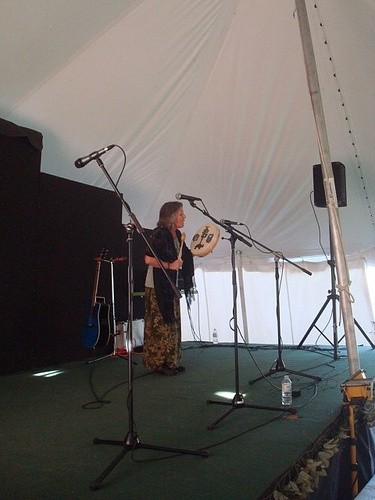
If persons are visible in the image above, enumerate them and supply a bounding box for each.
[143,201,186,376]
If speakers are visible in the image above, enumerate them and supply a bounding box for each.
[313,161,347,207]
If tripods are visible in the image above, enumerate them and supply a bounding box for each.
[91,157,375,492]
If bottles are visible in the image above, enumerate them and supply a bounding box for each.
[213,328,218,344]
[282,374,292,406]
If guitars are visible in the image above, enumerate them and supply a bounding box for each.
[82,247,110,349]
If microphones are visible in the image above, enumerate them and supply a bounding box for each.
[75,144,116,168]
[220,219,245,225]
[175,193,201,201]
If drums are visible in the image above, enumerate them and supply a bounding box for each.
[190,224,220,257]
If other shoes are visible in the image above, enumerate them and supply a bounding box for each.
[176,366,185,372]
[157,364,175,376]
